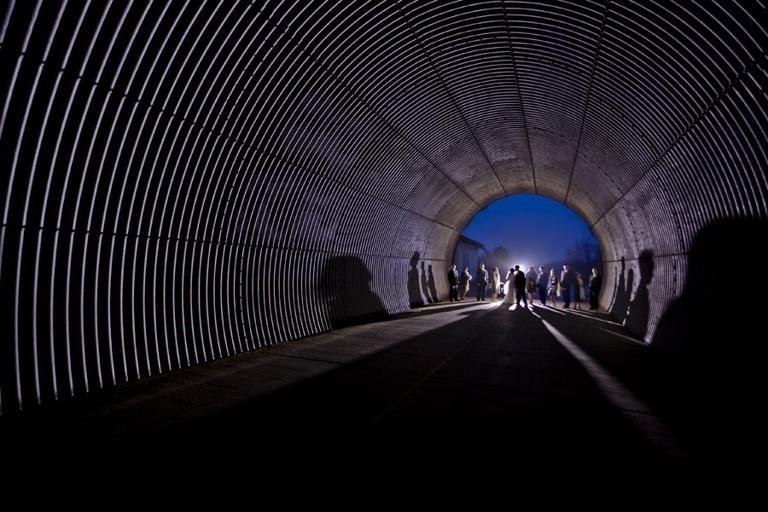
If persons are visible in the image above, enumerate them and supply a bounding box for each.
[448,262,602,311]
